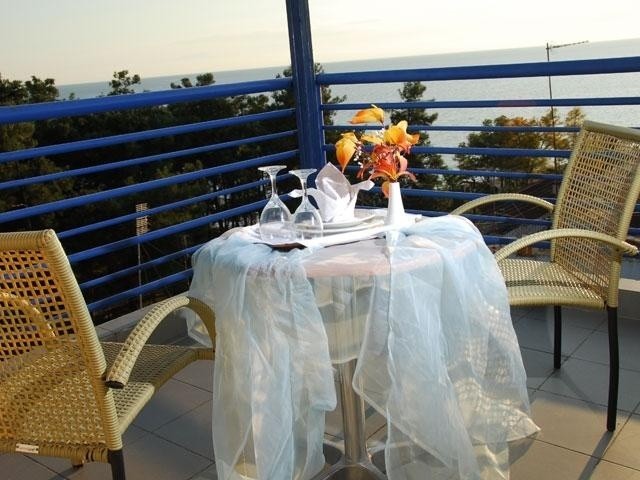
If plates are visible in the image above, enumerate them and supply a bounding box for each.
[296,210,377,228]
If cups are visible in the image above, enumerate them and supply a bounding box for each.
[288,168,323,241]
[257,165,293,242]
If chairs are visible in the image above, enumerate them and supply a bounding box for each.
[447,120,640,432]
[1,229,218,479]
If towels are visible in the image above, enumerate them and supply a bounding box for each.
[288,161,375,223]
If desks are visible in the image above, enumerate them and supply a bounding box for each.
[186,211,541,480]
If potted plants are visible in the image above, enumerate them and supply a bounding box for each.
[337,107,420,225]
